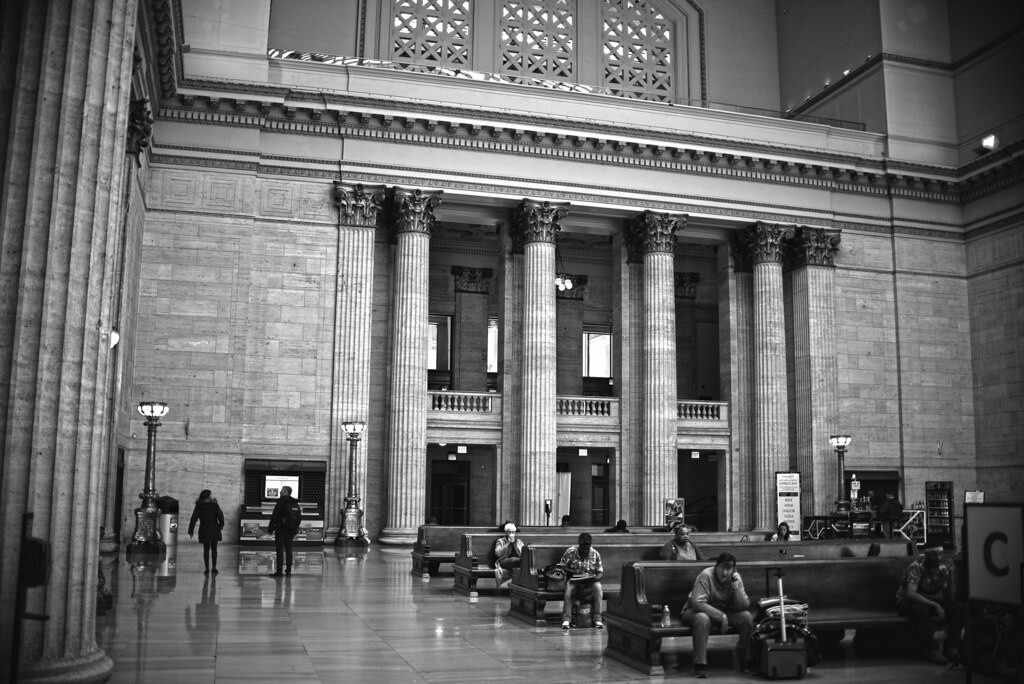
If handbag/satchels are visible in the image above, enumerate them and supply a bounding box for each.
[544,567,568,591]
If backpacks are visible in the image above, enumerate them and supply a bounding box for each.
[280,498,301,531]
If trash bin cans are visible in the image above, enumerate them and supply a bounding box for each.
[158,496,179,546]
[156,545,178,594]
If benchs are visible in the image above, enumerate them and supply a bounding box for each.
[410,524,960,674]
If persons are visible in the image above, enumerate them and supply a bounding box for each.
[494,523,525,588]
[770,523,792,542]
[681,553,760,679]
[659,522,706,560]
[560,533,605,629]
[604,520,630,533]
[896,540,967,667]
[878,490,903,540]
[268,486,301,577]
[426,511,439,526]
[188,490,225,575]
[560,515,572,526]
[499,520,520,532]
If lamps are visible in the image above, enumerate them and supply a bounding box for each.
[982,134,1001,151]
[556,242,573,291]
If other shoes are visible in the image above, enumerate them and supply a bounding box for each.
[286,571,292,577]
[204,569,209,574]
[946,648,959,661]
[211,568,218,573]
[270,571,283,577]
[926,649,947,665]
[561,620,570,629]
[592,621,604,628]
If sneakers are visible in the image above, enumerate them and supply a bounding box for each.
[692,664,709,678]
[742,660,760,675]
